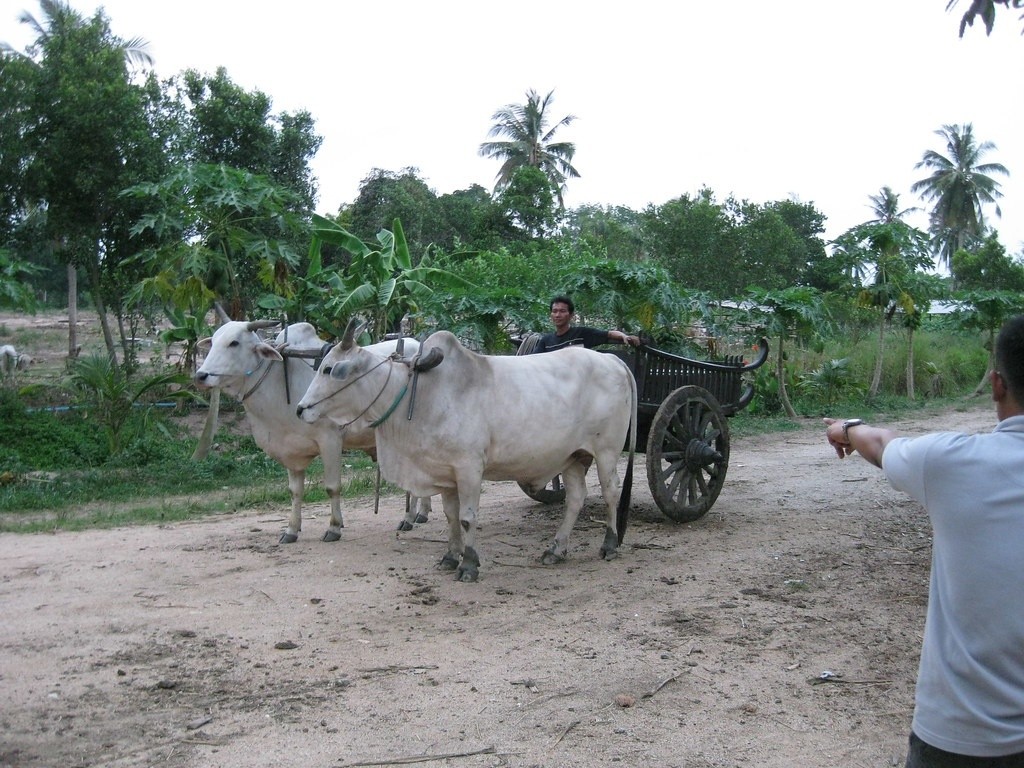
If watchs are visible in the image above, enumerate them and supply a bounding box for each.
[842,418,866,445]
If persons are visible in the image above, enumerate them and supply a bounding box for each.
[823,316,1024,768]
[533,297,640,354]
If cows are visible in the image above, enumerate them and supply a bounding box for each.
[193,299,640,586]
